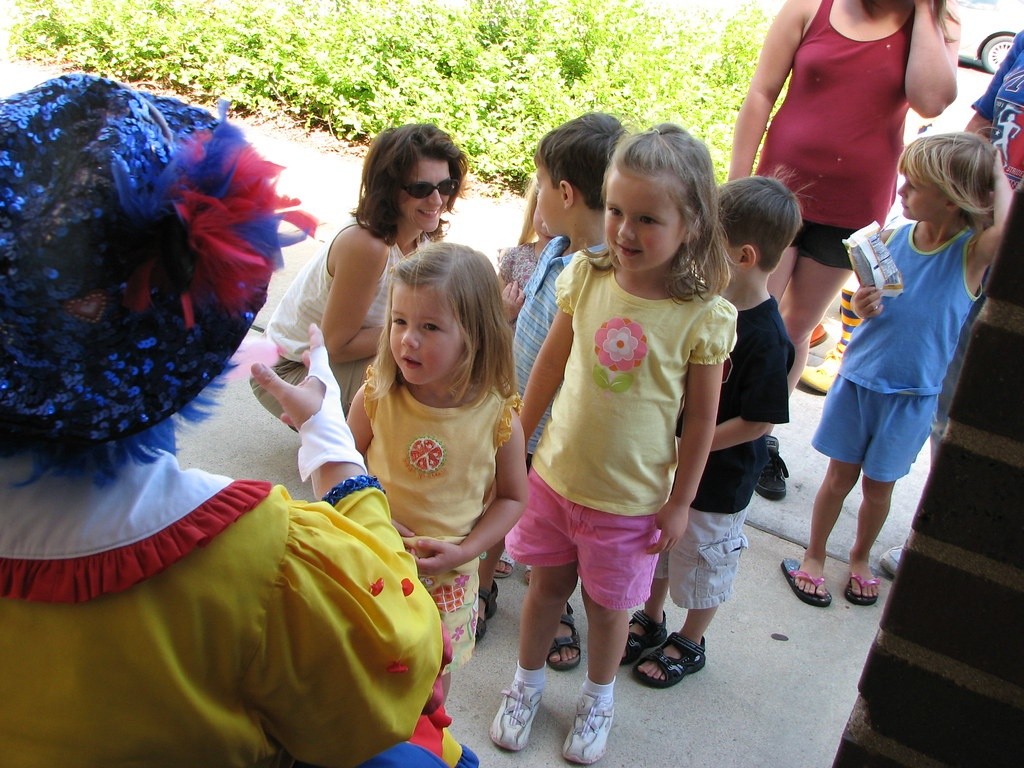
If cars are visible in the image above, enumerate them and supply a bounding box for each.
[953,0,1024,75]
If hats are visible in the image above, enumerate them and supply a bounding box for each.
[0,74,317,447]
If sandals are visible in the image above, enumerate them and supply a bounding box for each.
[544,600,580,671]
[633,632,705,689]
[618,609,667,667]
[524,564,534,584]
[492,546,516,578]
[474,579,498,645]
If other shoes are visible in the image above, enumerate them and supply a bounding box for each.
[880,544,903,577]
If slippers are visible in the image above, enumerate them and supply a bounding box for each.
[781,558,832,607]
[844,571,880,605]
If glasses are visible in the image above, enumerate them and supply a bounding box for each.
[400,178,459,199]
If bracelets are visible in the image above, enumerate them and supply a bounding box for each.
[322,475,387,507]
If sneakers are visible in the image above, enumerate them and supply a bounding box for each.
[561,684,614,763]
[799,349,843,394]
[755,435,789,500]
[490,680,544,751]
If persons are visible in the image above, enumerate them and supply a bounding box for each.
[879,29,1024,577]
[345,241,529,710]
[475,112,625,673]
[494,173,554,585]
[250,123,470,432]
[730,0,960,502]
[619,175,803,689]
[490,122,738,764]
[781,132,1013,608]
[0,73,483,768]
